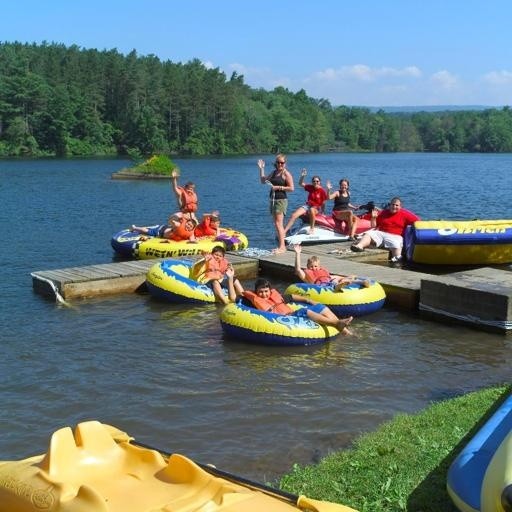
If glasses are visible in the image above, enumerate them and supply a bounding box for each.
[276,160,284,164]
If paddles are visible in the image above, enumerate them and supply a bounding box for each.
[130,440,357,512]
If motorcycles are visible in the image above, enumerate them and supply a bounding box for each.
[284,199,395,246]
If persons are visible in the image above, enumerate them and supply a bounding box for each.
[295,243,370,291]
[194,210,222,237]
[130,217,199,244]
[171,167,200,227]
[351,197,422,263]
[326,178,362,243]
[226,263,354,339]
[202,246,246,305]
[257,153,295,256]
[285,168,328,236]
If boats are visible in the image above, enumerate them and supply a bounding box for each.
[405,220,512,264]
[0,419,354,511]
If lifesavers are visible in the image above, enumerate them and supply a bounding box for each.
[146,260,238,305]
[220,301,339,347]
[111,224,248,259]
[283,275,387,318]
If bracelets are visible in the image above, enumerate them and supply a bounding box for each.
[282,186,283,191]
[279,186,281,191]
